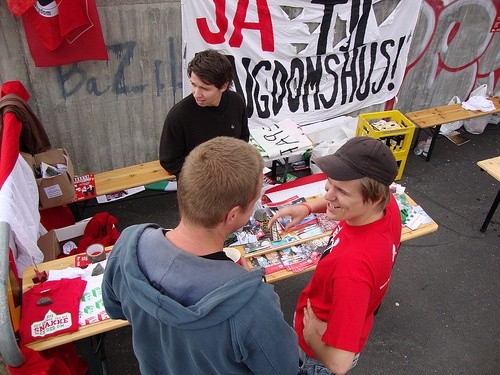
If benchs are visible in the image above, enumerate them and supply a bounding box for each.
[39,160,176,209]
[407,97,500,162]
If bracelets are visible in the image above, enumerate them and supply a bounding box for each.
[301,203,312,216]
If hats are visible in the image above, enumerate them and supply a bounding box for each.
[311,137,399,187]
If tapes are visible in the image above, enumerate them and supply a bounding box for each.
[85,243,106,263]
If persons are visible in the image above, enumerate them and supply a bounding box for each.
[268,136,402,375]
[315,240,328,254]
[101,137,300,375]
[253,255,271,268]
[159,50,251,188]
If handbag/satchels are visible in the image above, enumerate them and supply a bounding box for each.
[440,83,491,135]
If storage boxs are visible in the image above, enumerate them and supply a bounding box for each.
[355,110,416,180]
[37,217,121,263]
[20,148,75,209]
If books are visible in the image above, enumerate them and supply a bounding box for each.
[266,194,319,234]
[249,234,330,273]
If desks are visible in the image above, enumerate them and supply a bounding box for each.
[22,182,438,375]
[476,156,500,233]
